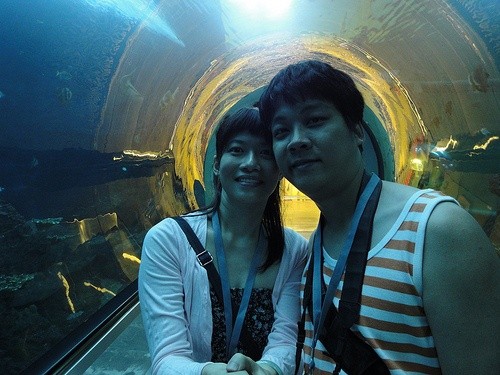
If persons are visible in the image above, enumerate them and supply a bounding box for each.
[257,59,500,375]
[137,107,313,375]
